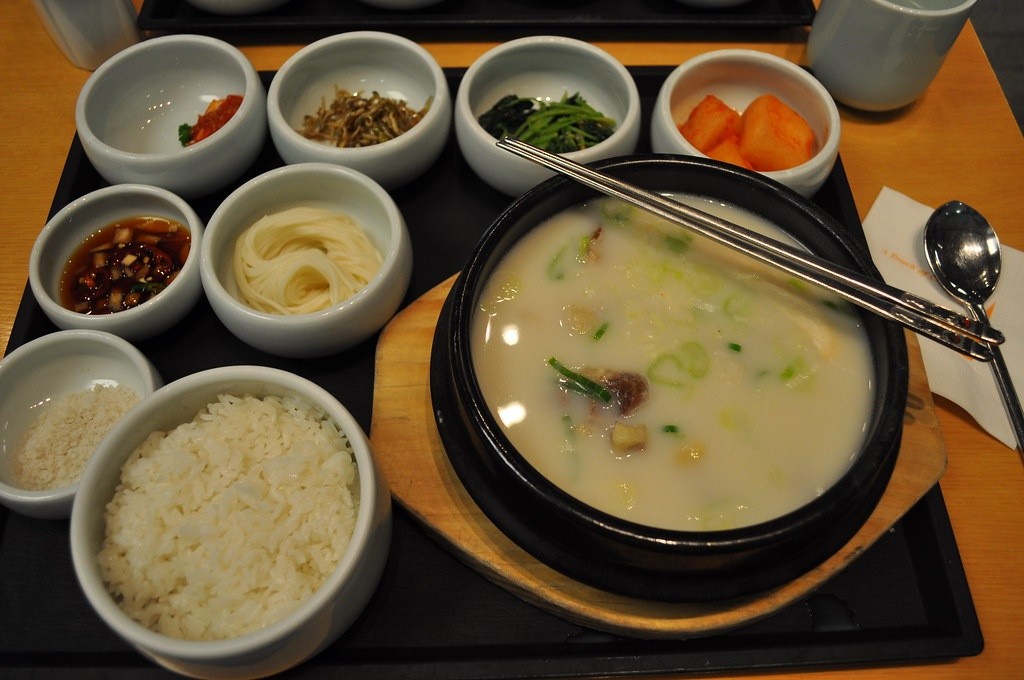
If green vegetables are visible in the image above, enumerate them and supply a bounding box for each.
[477,89,619,156]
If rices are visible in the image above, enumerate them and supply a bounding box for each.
[97,394,361,642]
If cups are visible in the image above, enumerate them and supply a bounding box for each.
[31,0,142,72]
[805,0,978,112]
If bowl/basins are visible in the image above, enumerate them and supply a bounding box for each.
[266,30,454,192]
[69,365,388,680]
[651,49,843,201]
[448,153,909,580]
[75,34,268,201]
[0,328,166,520]
[456,34,642,199]
[28,183,212,345]
[199,162,414,360]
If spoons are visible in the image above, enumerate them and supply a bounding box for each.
[923,200,1024,458]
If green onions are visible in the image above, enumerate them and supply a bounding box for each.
[548,198,813,437]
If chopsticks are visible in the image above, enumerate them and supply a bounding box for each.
[496,135,1006,363]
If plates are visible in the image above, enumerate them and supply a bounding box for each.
[430,273,902,602]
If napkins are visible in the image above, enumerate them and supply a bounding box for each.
[862,187,1024,450]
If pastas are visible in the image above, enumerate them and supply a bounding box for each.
[233,206,384,316]
